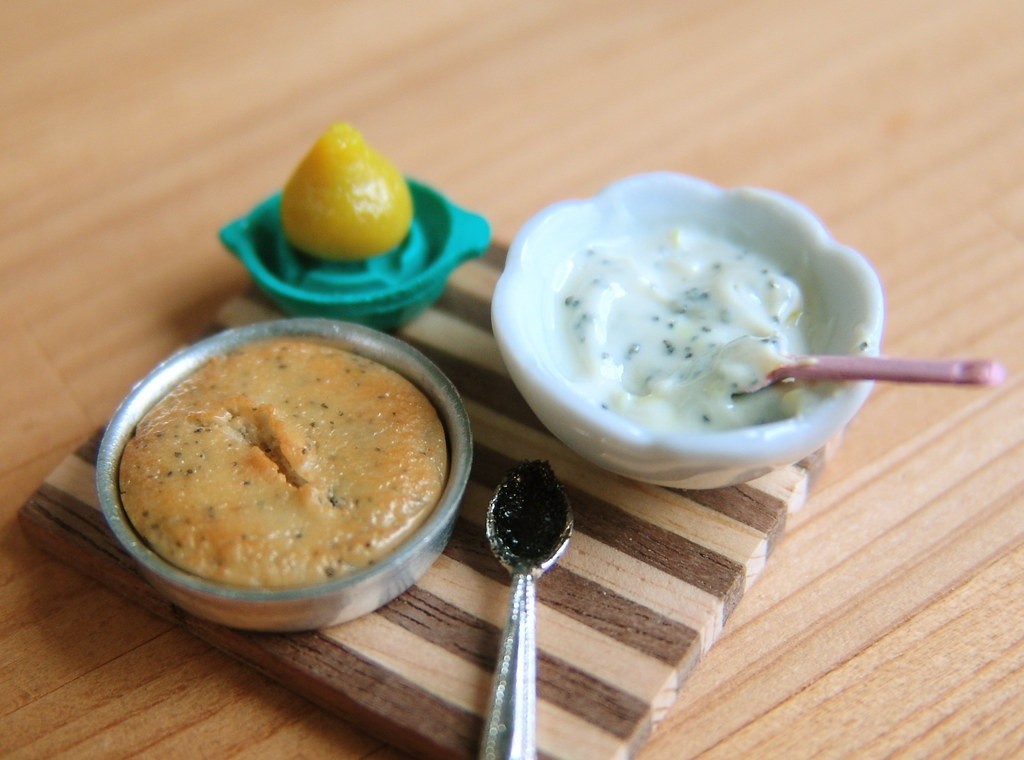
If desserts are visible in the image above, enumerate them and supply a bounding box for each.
[281,119,411,262]
[119,334,445,588]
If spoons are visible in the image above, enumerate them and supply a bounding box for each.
[475,460,573,759]
[704,336,1003,399]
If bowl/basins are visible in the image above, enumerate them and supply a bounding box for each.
[489,169,883,490]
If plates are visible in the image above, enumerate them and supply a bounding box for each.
[94,318,475,632]
